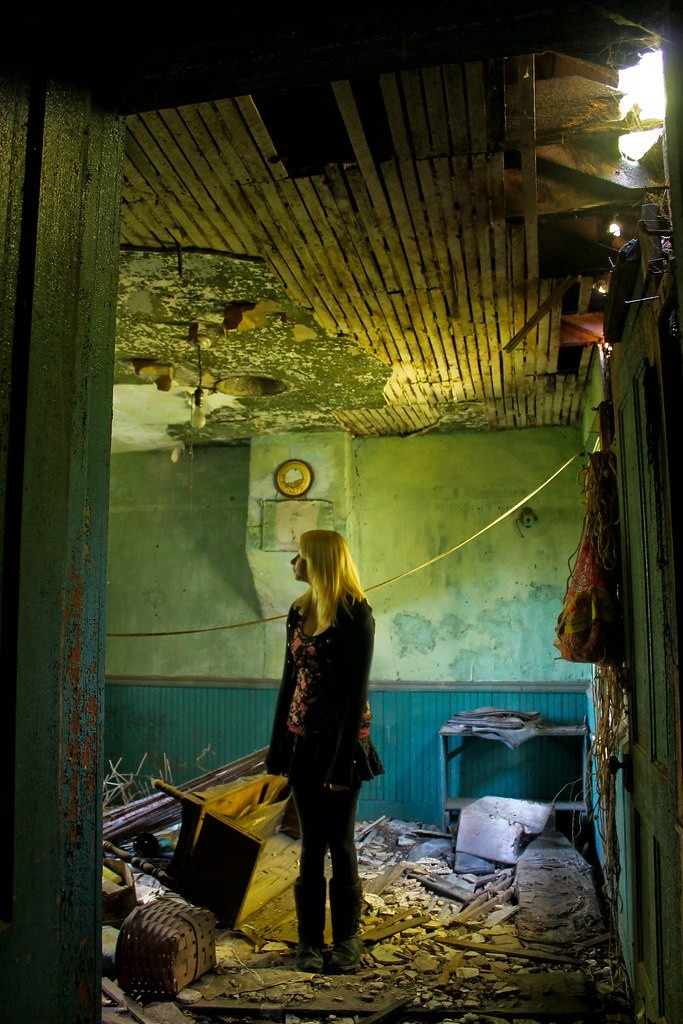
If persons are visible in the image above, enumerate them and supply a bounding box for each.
[264,530,384,973]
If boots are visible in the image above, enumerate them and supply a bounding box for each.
[294,877,326,973]
[328,876,362,969]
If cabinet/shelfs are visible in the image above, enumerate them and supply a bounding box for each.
[439,716,599,871]
[103,773,305,932]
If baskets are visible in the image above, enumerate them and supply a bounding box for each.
[115,894,217,1002]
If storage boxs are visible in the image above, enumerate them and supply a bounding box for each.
[102,858,138,926]
